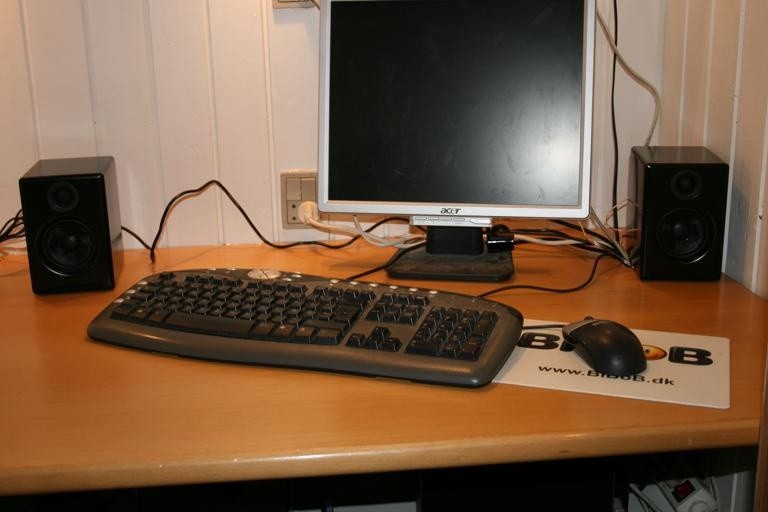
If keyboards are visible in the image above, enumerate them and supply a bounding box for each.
[83,265,525,389]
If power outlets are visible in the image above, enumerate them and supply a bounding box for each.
[281,199,323,231]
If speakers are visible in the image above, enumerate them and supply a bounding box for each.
[624,145,731,282]
[17,155,125,296]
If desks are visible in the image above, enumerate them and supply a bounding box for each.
[0,227,766,509]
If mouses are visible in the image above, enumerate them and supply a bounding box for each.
[561,315,648,377]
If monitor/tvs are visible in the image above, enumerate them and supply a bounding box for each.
[316,0,598,283]
